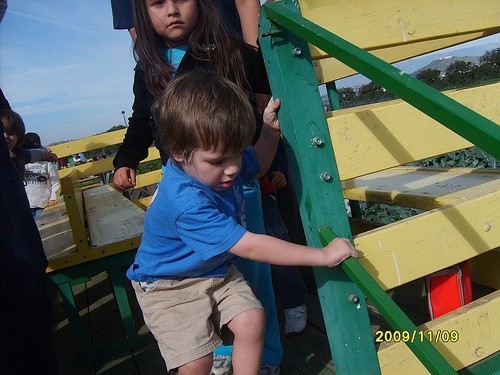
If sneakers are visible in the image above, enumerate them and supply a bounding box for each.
[258,364,280,375]
[284,304,308,336]
[209,354,233,375]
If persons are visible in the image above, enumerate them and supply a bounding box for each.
[23,131,62,220]
[0,118,52,375]
[111,0,315,292]
[112,0,308,335]
[128,67,360,375]
[0,108,59,171]
[72,153,109,182]
